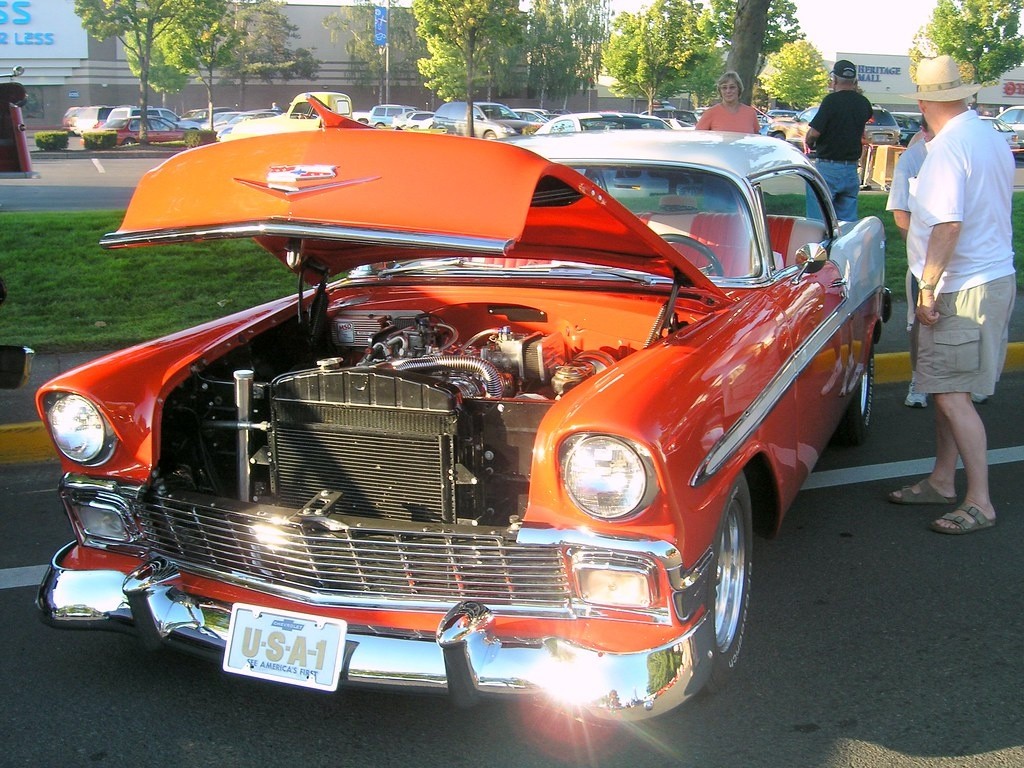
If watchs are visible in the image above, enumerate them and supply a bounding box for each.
[918,280,936,290]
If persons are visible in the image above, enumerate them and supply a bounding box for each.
[888,56,1016,533]
[803,60,872,223]
[272,102,281,110]
[695,72,760,134]
[886,114,987,407]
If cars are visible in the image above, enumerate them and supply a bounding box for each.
[35,94,892,724]
[62,92,1024,157]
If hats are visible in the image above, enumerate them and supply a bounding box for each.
[829,60,857,78]
[899,55,983,102]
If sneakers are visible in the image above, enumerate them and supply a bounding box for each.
[972,392,987,403]
[904,380,928,407]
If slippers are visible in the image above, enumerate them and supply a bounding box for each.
[888,480,958,504]
[929,503,996,533]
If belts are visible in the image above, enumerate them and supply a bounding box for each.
[817,158,860,166]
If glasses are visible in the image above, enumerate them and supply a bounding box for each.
[719,85,739,90]
[830,74,836,80]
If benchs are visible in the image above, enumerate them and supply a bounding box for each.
[472,211,827,279]
